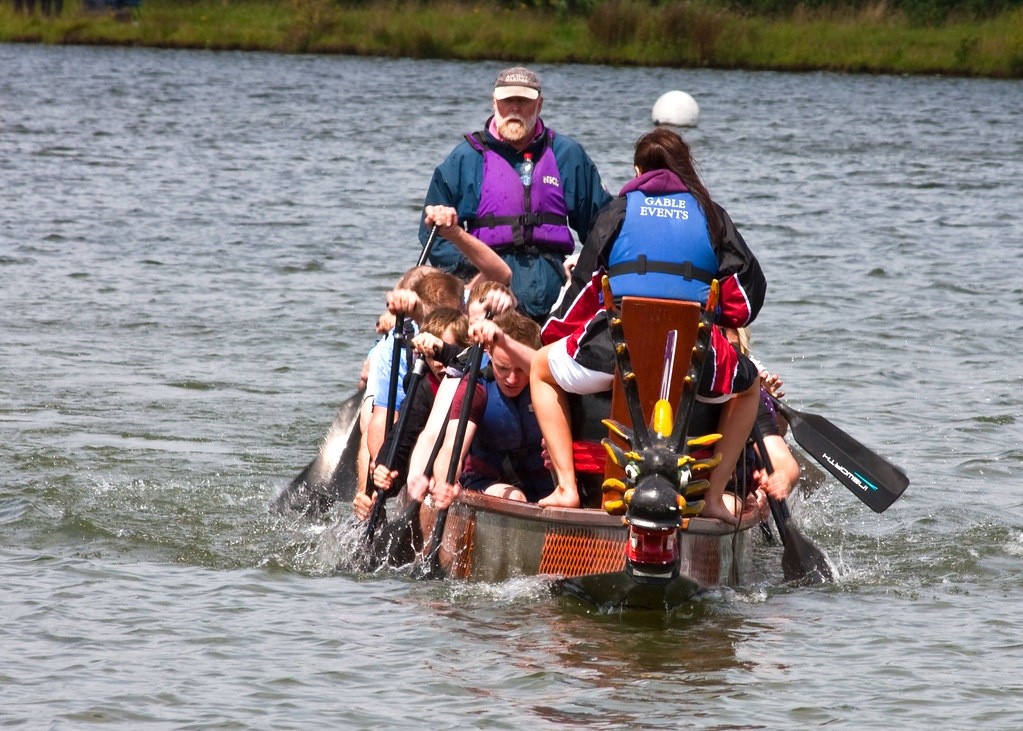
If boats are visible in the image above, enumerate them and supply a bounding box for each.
[396,475,772,595]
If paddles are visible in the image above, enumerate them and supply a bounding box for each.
[408,295,494,584]
[269,453,336,524]
[298,222,442,504]
[335,337,437,574]
[364,304,493,576]
[762,384,914,517]
[381,300,405,444]
[752,419,837,588]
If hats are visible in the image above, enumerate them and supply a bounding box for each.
[491,67,538,102]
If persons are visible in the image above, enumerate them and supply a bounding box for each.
[529,128,767,509]
[418,66,616,324]
[354,205,801,522]
